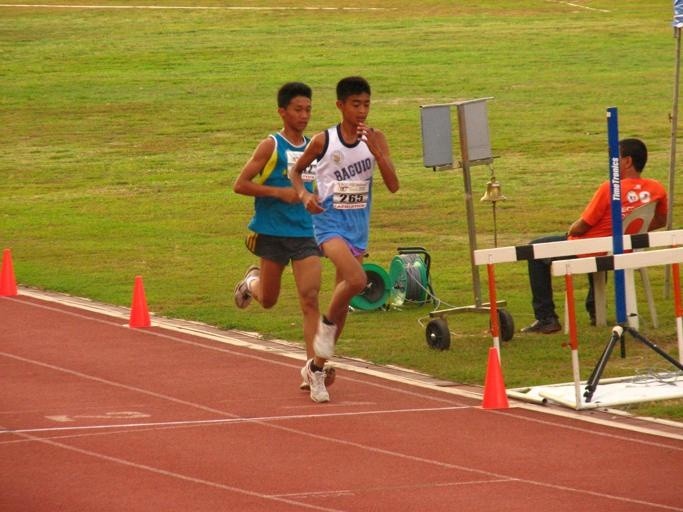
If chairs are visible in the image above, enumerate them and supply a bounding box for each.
[565,202,659,335]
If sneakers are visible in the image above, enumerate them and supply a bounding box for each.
[299,359,336,403]
[520,318,561,334]
[234,265,259,309]
[313,318,337,360]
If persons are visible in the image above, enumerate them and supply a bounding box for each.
[287,76,399,403]
[519,139,667,336]
[231,82,337,391]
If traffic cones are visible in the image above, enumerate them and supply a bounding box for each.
[483,346,510,411]
[128,275,152,329]
[0,247,18,297]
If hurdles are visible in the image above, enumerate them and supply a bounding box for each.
[473,229,683,411]
